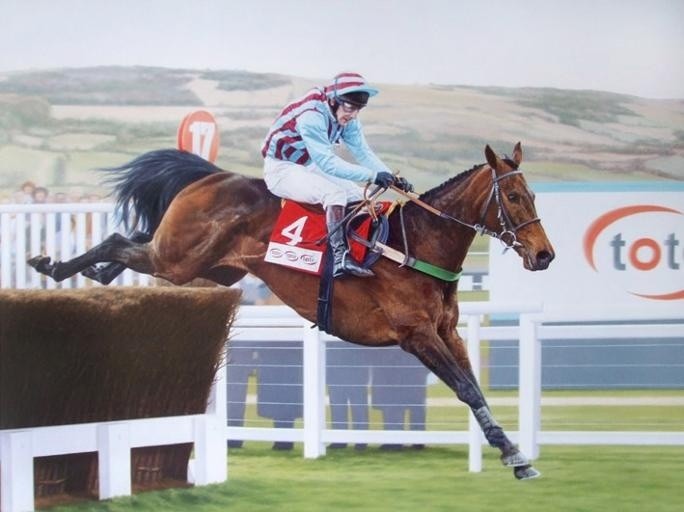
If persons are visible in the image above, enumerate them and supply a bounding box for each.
[227,274,433,452]
[262,68,415,279]
[0,178,140,289]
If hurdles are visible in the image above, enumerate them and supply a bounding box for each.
[1,287,242,511]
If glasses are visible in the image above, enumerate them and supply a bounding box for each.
[335,95,367,112]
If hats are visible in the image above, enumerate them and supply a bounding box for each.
[323,68,379,97]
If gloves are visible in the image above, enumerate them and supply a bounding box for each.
[375,171,416,195]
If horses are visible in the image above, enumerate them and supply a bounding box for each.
[26,140,556,481]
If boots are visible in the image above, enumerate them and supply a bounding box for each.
[326,205,375,279]
